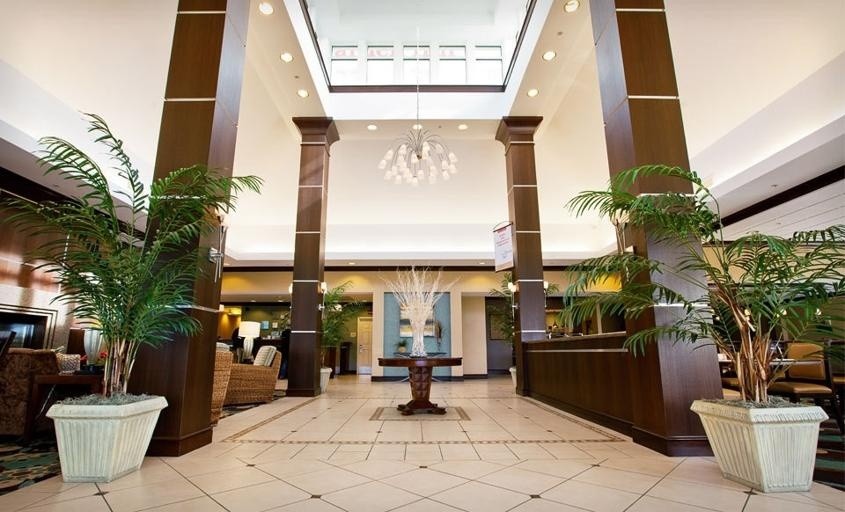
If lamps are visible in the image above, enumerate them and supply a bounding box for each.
[611,204,635,282]
[289,284,292,311]
[318,283,329,319]
[544,280,549,311]
[238,321,261,362]
[209,202,234,282]
[508,282,518,320]
[378,27,458,187]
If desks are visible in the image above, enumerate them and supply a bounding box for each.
[394,352,447,382]
[378,358,462,415]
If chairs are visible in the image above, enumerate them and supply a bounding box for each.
[721,339,845,441]
[1,341,281,441]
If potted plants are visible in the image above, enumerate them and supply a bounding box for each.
[397,338,408,352]
[279,282,364,391]
[557,165,845,492]
[490,273,559,390]
[0,110,262,482]
